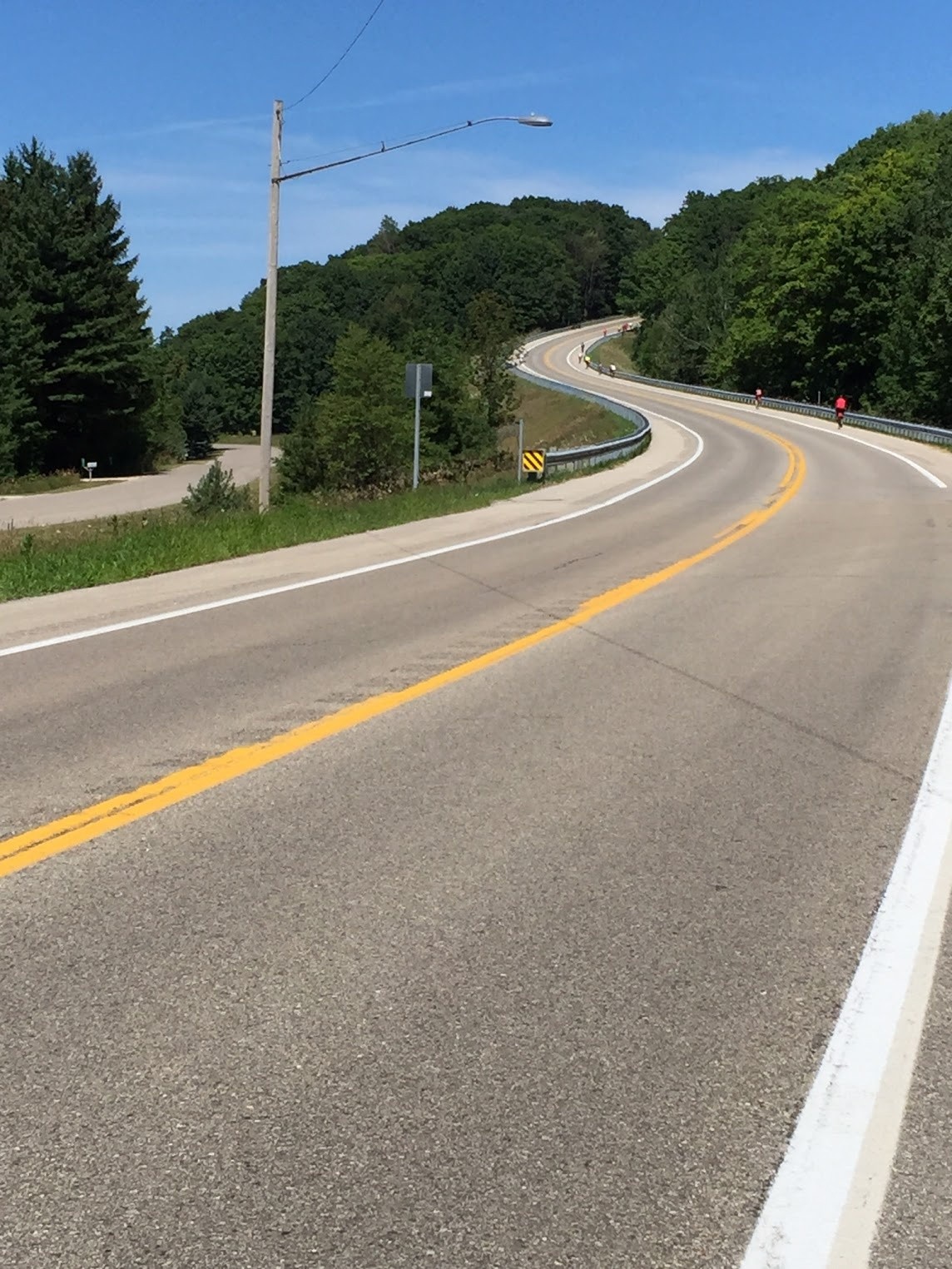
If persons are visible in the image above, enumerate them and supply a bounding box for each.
[834,394,847,420]
[602,328,607,337]
[755,387,762,400]
[581,342,585,353]
[584,356,591,365]
[617,322,633,333]
[598,361,603,372]
[609,363,616,373]
[579,353,583,361]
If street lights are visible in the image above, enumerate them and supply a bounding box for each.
[258,113,555,515]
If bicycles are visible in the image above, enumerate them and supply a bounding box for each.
[755,396,760,408]
[837,410,843,429]
